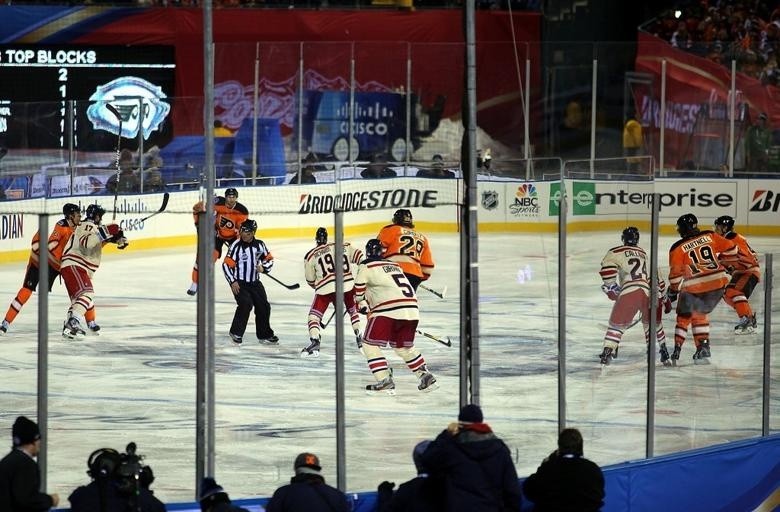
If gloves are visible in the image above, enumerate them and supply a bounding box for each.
[662,297,672,314]
[356,300,370,314]
[602,283,622,300]
[98,223,129,249]
[667,287,679,302]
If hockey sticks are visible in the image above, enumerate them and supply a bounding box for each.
[101,193,169,242]
[320,310,335,328]
[265,273,299,289]
[599,316,642,332]
[419,285,447,299]
[414,330,451,347]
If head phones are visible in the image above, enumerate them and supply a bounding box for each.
[86,447,123,482]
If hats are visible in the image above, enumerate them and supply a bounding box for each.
[12,416,40,446]
[294,452,322,472]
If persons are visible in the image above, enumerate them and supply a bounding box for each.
[375,439,438,511]
[263,452,347,509]
[301,225,363,355]
[423,403,523,511]
[653,0,780,89]
[359,154,396,178]
[599,226,672,366]
[1,201,102,334]
[351,239,438,391]
[286,158,319,185]
[665,213,741,362]
[744,111,770,174]
[520,428,607,511]
[763,115,779,172]
[222,217,279,343]
[476,148,494,173]
[59,204,129,336]
[102,144,164,193]
[184,184,247,298]
[66,447,166,510]
[213,120,234,138]
[623,108,643,173]
[374,208,438,296]
[1,414,60,512]
[415,153,455,179]
[714,215,763,338]
[196,476,253,511]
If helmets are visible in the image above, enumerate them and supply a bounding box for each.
[366,239,383,257]
[225,189,238,198]
[677,213,697,237]
[63,203,79,214]
[395,209,412,225]
[86,204,104,219]
[315,228,327,244]
[239,219,257,232]
[623,227,639,245]
[715,216,734,230]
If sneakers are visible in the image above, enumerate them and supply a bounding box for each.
[87,320,100,331]
[187,282,197,295]
[268,335,278,342]
[62,318,85,335]
[0,326,7,336]
[229,332,242,343]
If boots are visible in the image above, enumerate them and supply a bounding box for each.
[418,365,435,390]
[692,339,710,359]
[735,312,757,329]
[366,368,395,390]
[671,346,682,359]
[601,348,613,363]
[302,334,322,353]
[659,344,669,362]
